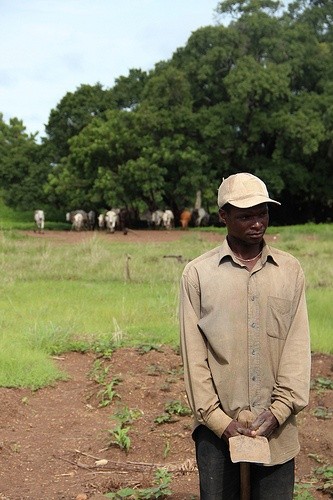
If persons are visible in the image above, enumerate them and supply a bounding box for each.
[175,172,313,500]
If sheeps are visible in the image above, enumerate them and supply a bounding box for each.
[34,209,46,235]
[65,208,226,234]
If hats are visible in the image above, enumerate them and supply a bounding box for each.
[217,173,280,209]
